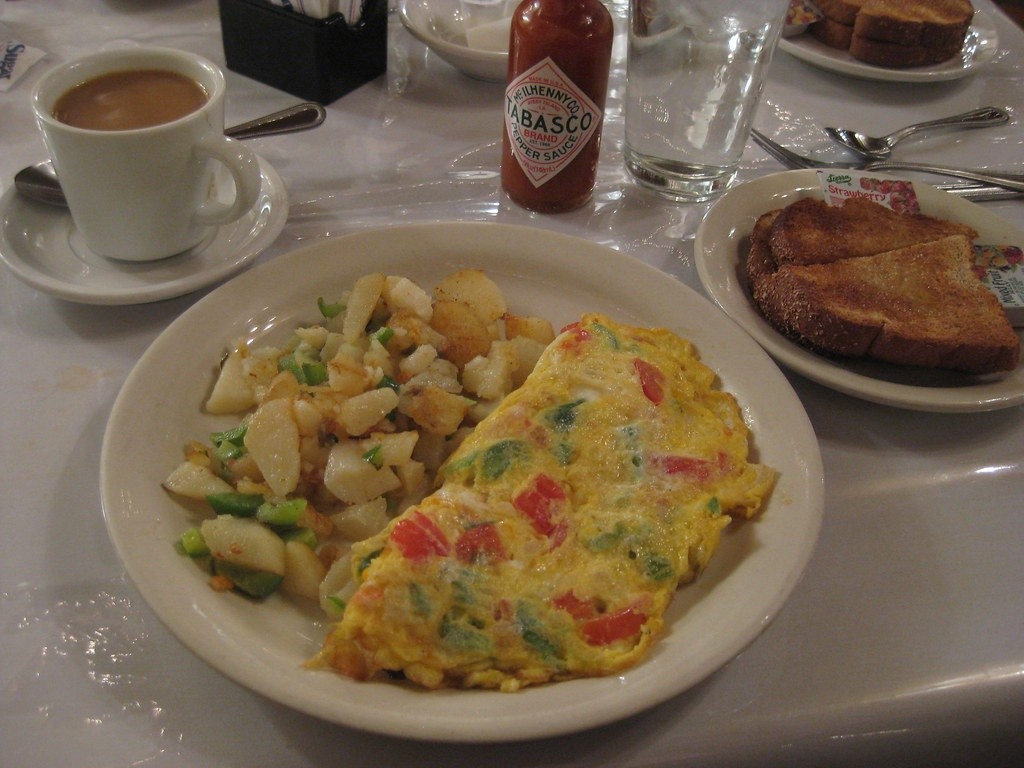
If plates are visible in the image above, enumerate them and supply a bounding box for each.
[0,134,289,305]
[100,222,823,740]
[694,169,1024,412]
[397,0,524,83]
[778,0,999,82]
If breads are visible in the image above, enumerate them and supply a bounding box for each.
[805,0,974,67]
[743,196,1018,377]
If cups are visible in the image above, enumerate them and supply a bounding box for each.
[625,0,790,202]
[30,46,261,261]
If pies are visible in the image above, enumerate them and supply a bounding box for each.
[292,309,780,690]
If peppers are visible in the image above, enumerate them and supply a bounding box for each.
[178,293,398,613]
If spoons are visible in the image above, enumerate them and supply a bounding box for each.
[825,106,1009,159]
[15,100,327,206]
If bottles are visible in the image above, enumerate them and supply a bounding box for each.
[501,0,614,215]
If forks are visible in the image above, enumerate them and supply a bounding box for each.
[749,128,1024,192]
